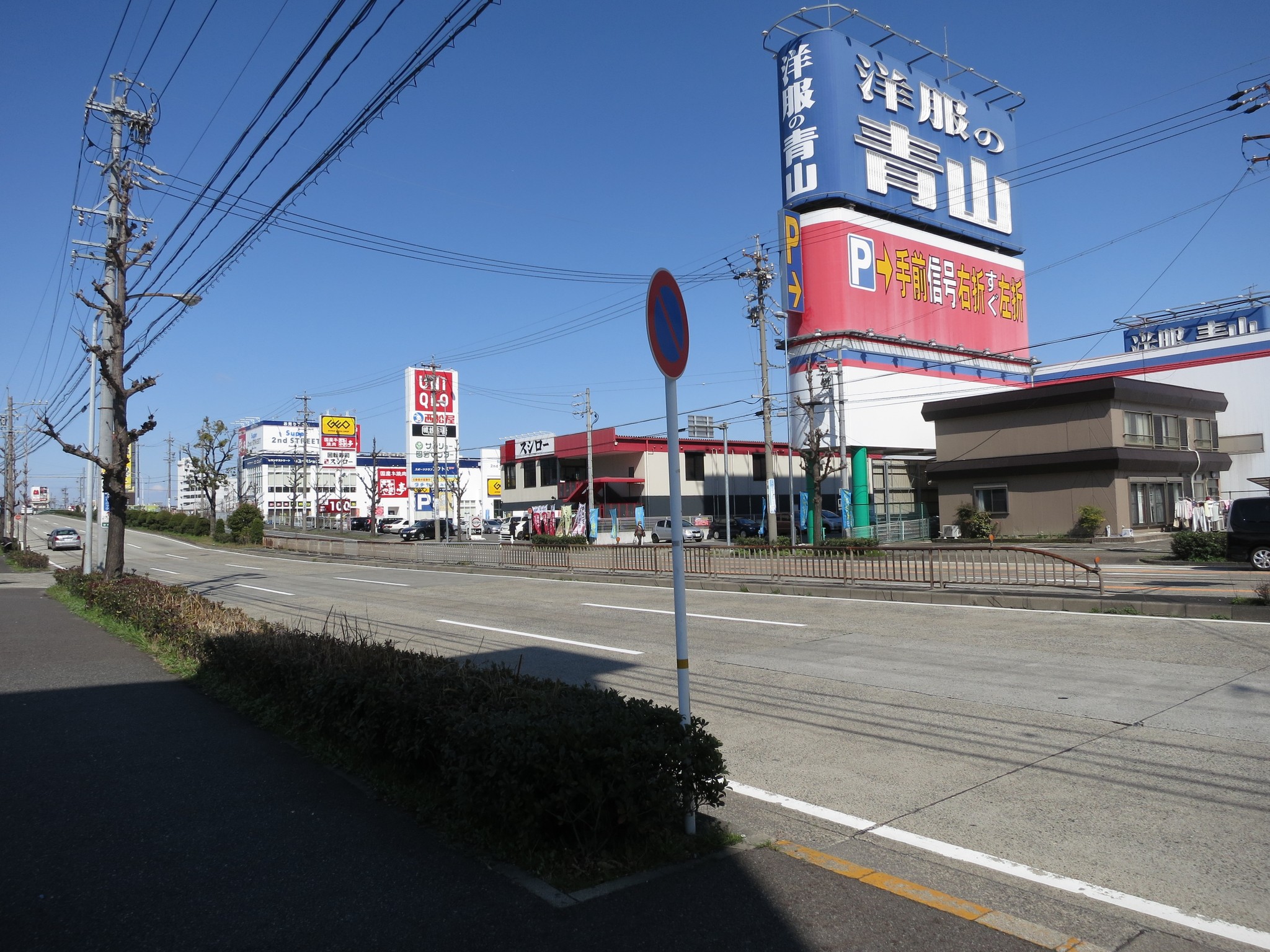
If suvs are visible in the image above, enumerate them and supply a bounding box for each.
[708,516,767,540]
[499,517,523,539]
[521,517,563,540]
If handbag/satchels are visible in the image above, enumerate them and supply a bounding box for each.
[642,531,646,537]
[633,536,637,544]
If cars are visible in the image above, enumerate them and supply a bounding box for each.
[266,520,273,525]
[47,528,82,551]
[483,518,503,535]
[651,518,706,543]
[512,519,525,541]
[400,519,454,540]
[767,512,809,536]
[448,521,463,536]
[461,520,471,534]
[325,515,419,534]
[820,509,845,534]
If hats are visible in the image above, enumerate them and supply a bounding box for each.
[638,521,642,524]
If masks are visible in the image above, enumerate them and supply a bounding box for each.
[639,524,641,525]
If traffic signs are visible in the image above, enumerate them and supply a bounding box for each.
[778,207,807,315]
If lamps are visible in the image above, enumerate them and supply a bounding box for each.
[928,338,936,346]
[1030,356,1037,362]
[761,7,1022,114]
[774,338,781,347]
[866,328,874,336]
[815,328,823,336]
[898,333,906,341]
[957,343,964,350]
[983,348,990,354]
[1007,352,1014,359]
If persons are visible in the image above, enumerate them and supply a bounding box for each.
[634,521,646,549]
[0,537,12,553]
[521,521,529,541]
[509,520,516,540]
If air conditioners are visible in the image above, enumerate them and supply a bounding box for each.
[943,525,961,537]
[1212,518,1224,531]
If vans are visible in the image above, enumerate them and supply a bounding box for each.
[21,504,34,515]
[1223,496,1270,571]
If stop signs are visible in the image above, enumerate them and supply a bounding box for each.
[15,515,21,520]
[527,508,533,514]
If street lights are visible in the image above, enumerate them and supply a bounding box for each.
[81,291,205,580]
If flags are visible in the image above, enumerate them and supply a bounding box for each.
[549,503,555,536]
[555,505,566,538]
[540,504,548,535]
[609,508,617,539]
[840,488,854,528]
[562,504,572,537]
[758,497,766,534]
[531,505,542,534]
[635,506,644,530]
[589,508,598,538]
[800,491,808,530]
[571,502,587,538]
